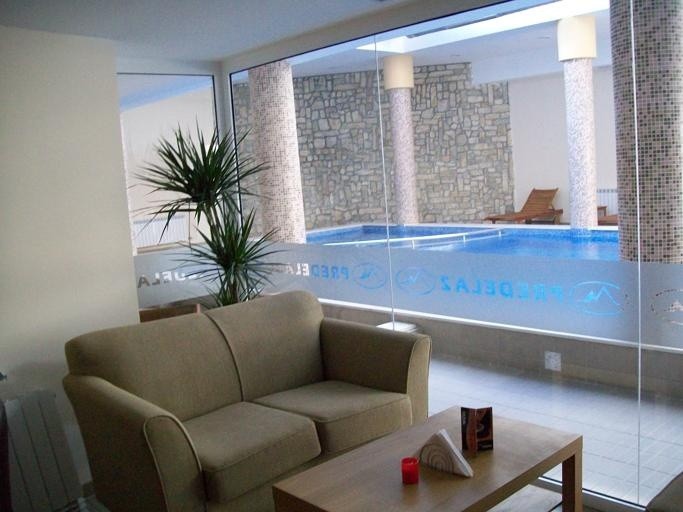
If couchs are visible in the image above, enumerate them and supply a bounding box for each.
[61,289,433,512]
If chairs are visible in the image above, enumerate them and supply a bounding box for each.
[483,187,564,225]
[598,214,619,226]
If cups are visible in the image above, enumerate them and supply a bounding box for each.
[400,454,420,484]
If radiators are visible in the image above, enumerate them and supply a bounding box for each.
[3,389,83,512]
